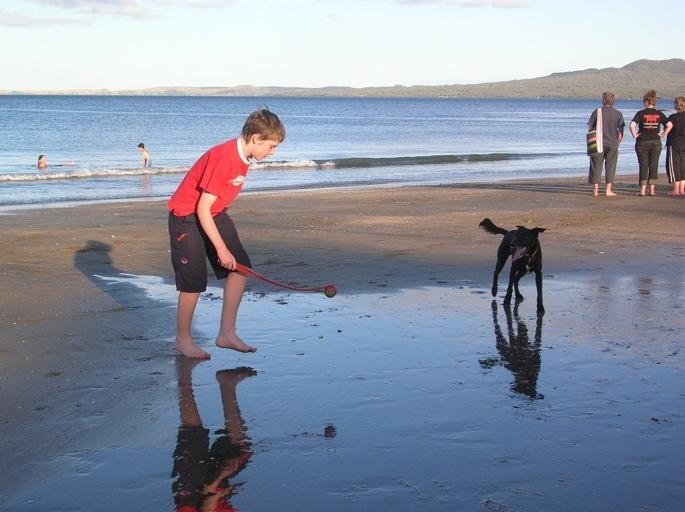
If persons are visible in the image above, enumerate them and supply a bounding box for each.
[167,108,287,360]
[587,91,624,198]
[38,155,47,168]
[629,89,674,196]
[173,356,261,512]
[667,96,685,196]
[137,143,152,168]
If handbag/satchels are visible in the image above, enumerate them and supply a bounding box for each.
[586,130,604,157]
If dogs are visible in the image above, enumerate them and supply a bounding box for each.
[478,217,548,311]
[480,300,545,401]
